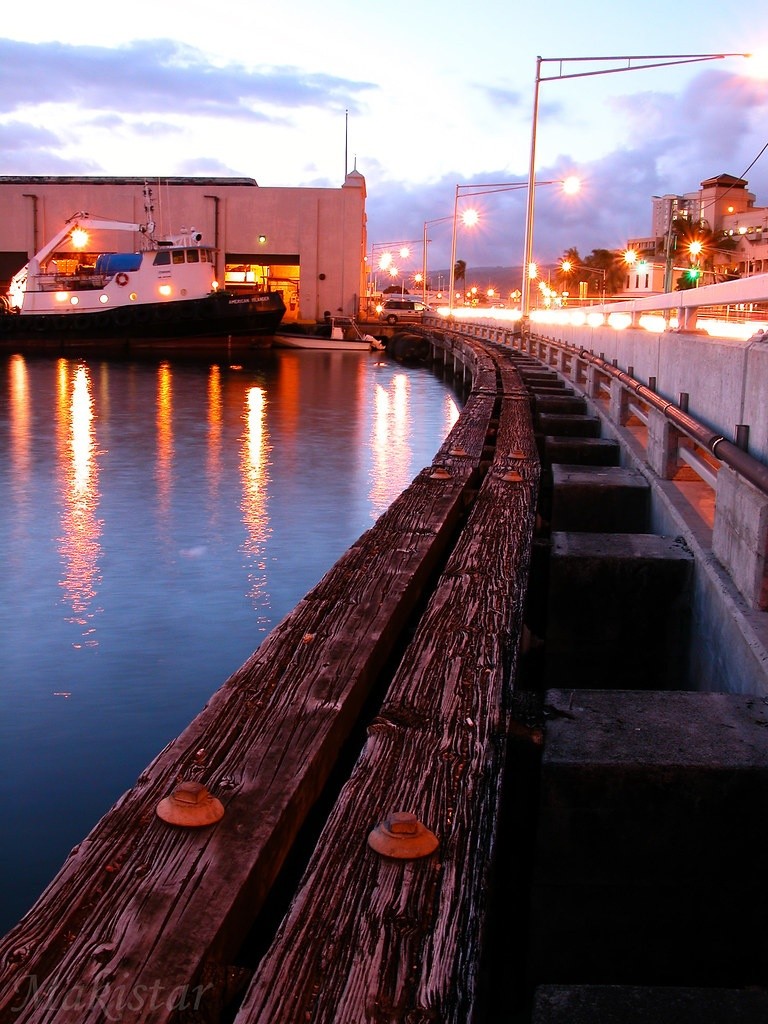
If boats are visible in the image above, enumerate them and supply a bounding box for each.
[385,331,429,368]
[273,315,373,350]
[3,212,287,354]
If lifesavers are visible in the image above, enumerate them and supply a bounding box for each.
[116,273,128,286]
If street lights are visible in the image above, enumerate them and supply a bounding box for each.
[625,251,666,290]
[690,242,750,278]
[488,287,494,303]
[369,240,432,296]
[414,274,422,291]
[449,178,580,315]
[422,210,478,304]
[562,262,605,298]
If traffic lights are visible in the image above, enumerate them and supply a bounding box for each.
[689,269,699,279]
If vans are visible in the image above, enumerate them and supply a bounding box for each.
[378,299,444,324]
